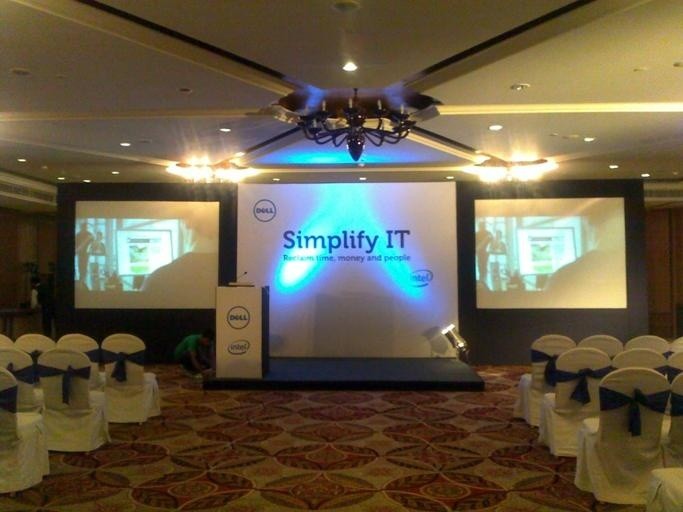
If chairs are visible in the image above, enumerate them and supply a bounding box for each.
[514,329,682,511]
[0,330,164,494]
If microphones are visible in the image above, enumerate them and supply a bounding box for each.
[234,272,247,282]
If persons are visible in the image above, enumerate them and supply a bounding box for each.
[74,222,92,281]
[484,229,507,288]
[139,204,218,291]
[474,221,493,281]
[172,329,216,377]
[87,232,106,287]
[540,198,626,293]
[28,275,55,337]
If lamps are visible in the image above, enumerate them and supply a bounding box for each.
[267,88,440,162]
